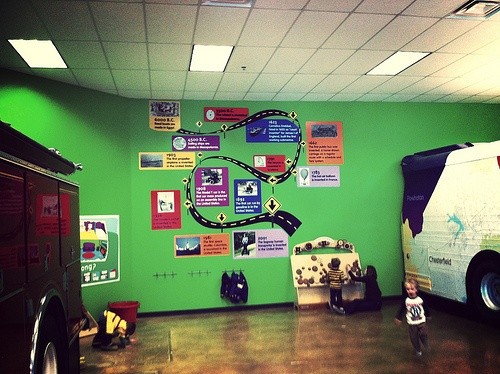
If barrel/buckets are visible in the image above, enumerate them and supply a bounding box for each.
[107,301,139,323]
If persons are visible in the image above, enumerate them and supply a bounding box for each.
[346,264,382,312]
[92,310,137,350]
[394,277,432,357]
[325,257,348,312]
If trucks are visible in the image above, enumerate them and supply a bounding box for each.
[399,140,500,328]
[0,119,88,374]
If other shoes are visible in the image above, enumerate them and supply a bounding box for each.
[329,301,335,312]
[93,342,101,347]
[103,341,119,349]
[333,305,346,315]
